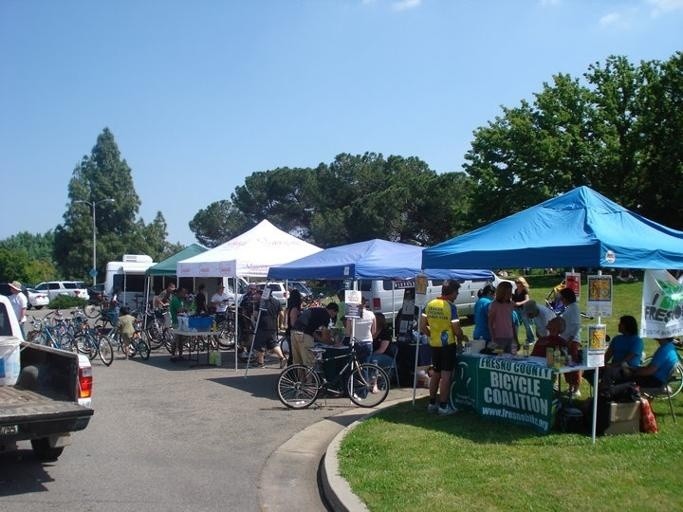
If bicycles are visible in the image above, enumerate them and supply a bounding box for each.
[637,355,682,401]
[273,336,390,414]
[26,291,237,367]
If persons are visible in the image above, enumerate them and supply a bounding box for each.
[196,283,287,370]
[287,289,394,393]
[6,280,28,342]
[422,279,679,432]
[117,306,139,360]
[158,283,190,361]
[111,288,122,306]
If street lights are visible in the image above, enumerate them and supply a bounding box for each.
[72,198,119,285]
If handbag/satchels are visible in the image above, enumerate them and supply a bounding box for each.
[639,397,659,434]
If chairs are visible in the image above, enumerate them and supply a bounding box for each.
[381,345,400,388]
[640,356,682,423]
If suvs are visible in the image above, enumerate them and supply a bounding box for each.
[34,279,90,300]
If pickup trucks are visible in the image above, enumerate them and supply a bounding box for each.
[0,292,96,468]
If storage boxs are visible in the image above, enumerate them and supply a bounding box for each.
[177,316,188,331]
[609,399,638,422]
[187,315,214,332]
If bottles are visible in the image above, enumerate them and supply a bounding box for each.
[510,336,517,357]
[521,338,528,358]
[478,335,484,349]
[545,343,553,368]
[208,349,221,366]
[553,343,559,369]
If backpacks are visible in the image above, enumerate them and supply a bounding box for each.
[258,312,276,337]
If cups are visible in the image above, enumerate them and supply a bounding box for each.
[470,340,480,354]
[494,344,504,356]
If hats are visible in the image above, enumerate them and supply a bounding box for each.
[522,300,538,313]
[8,280,23,293]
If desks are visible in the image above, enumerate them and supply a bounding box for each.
[452,344,598,428]
[394,342,433,385]
[169,329,221,368]
[298,342,349,391]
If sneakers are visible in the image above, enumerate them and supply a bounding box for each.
[565,387,582,398]
[526,337,537,346]
[168,355,177,361]
[179,355,188,361]
[427,401,439,414]
[253,362,266,369]
[279,356,288,368]
[438,401,456,416]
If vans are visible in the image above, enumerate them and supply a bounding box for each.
[360,267,519,327]
[104,254,248,322]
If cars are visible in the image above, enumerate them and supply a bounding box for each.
[90,283,104,300]
[246,280,313,305]
[23,287,51,311]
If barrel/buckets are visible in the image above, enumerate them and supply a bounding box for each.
[1,334,20,386]
[208,351,224,365]
